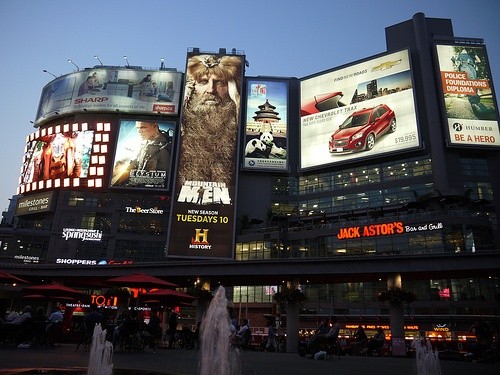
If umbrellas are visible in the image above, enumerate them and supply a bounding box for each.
[0,270,199,309]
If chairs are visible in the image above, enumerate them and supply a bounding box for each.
[0,318,387,360]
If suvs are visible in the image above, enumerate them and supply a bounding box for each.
[328,103,396,155]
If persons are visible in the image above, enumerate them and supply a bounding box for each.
[113,121,172,187]
[82,301,181,352]
[0,301,63,348]
[227,317,249,351]
[139,74,160,97]
[264,317,385,359]
[77,72,102,96]
[178,55,239,180]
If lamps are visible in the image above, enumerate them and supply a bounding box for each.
[68,59,79,71]
[123,56,129,67]
[43,70,57,79]
[161,58,165,70]
[94,56,103,67]
